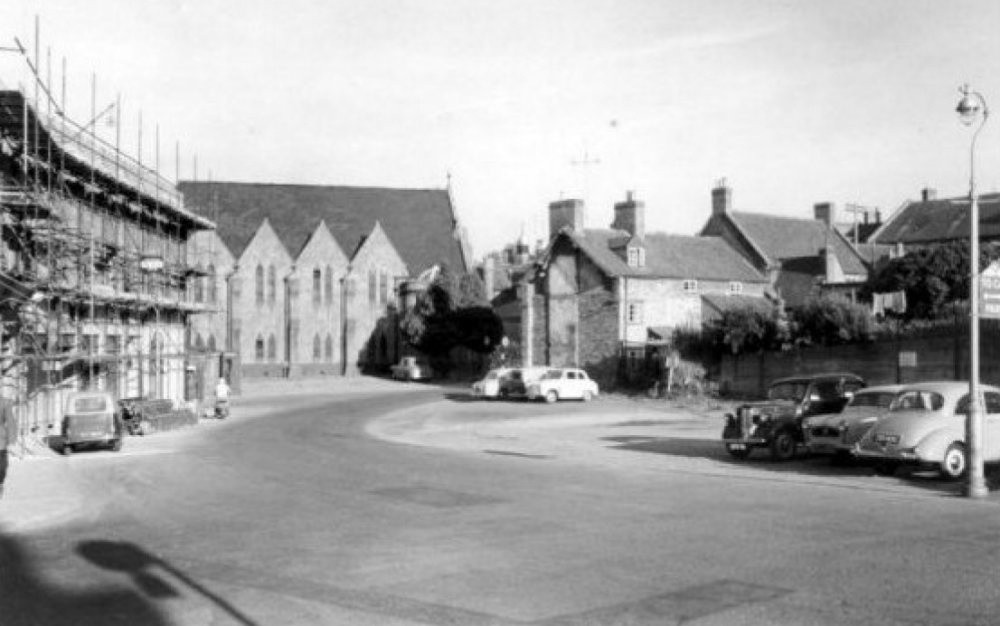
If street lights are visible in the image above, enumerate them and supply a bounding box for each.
[956,81,991,493]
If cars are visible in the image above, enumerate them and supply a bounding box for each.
[721,373,868,461]
[801,385,906,468]
[523,367,599,403]
[390,356,434,380]
[472,367,532,400]
[850,380,1000,481]
[63,392,122,452]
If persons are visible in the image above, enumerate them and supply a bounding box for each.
[214,377,231,416]
[647,348,660,388]
